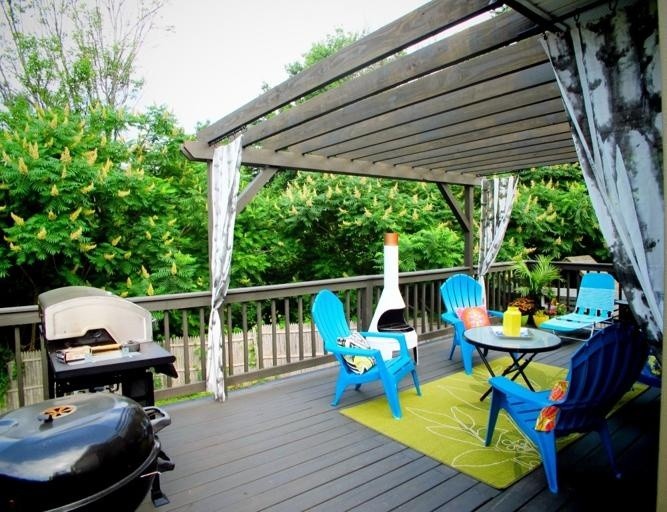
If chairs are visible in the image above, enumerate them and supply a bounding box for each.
[439,273,519,375]
[487,320,650,499]
[539,273,616,343]
[313,289,420,418]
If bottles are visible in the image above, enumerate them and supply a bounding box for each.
[503,307,522,337]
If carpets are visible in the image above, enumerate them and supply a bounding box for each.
[338,355,650,491]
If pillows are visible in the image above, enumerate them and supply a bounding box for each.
[455,305,491,331]
[534,379,568,432]
[336,330,377,375]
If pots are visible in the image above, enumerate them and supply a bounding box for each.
[0,392,176,512]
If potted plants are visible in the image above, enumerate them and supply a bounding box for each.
[512,255,565,330]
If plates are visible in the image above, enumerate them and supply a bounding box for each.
[492,325,532,341]
